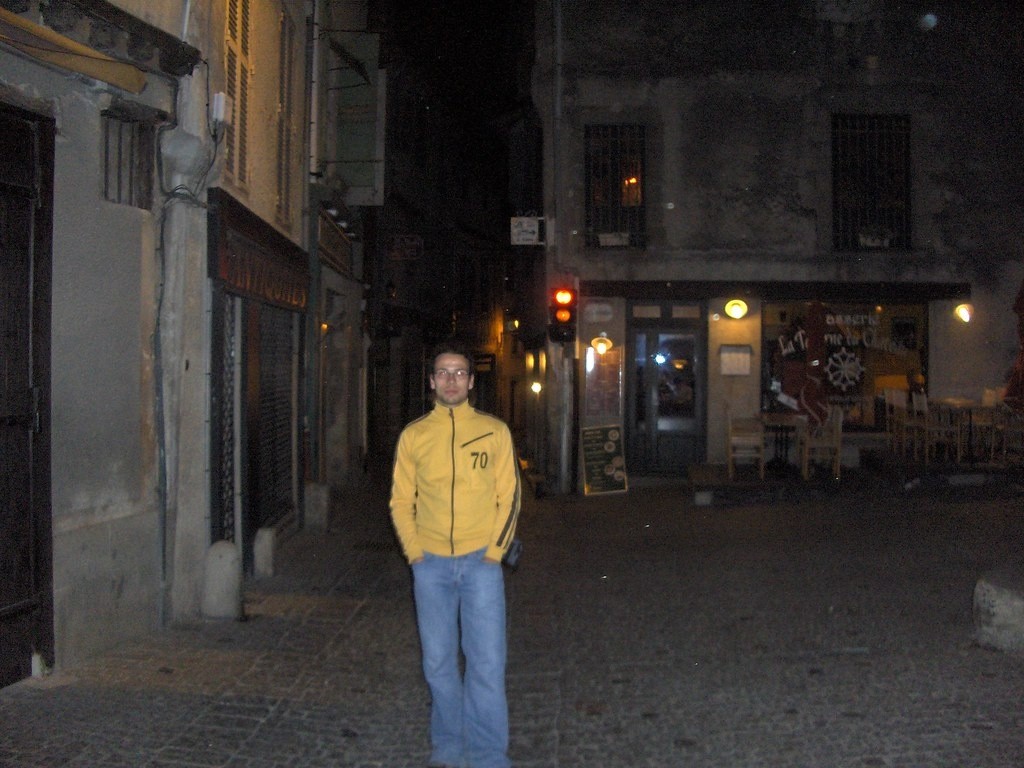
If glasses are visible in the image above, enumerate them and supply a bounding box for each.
[434,370,472,380]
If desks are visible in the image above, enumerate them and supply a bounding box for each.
[764,425,796,469]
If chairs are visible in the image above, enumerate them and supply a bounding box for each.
[882,386,955,463]
[726,405,764,478]
[958,397,1019,459]
[795,406,844,480]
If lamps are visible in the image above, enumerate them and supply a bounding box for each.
[590,336,614,355]
[724,299,748,320]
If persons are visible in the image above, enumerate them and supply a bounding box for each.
[387,342,523,768]
